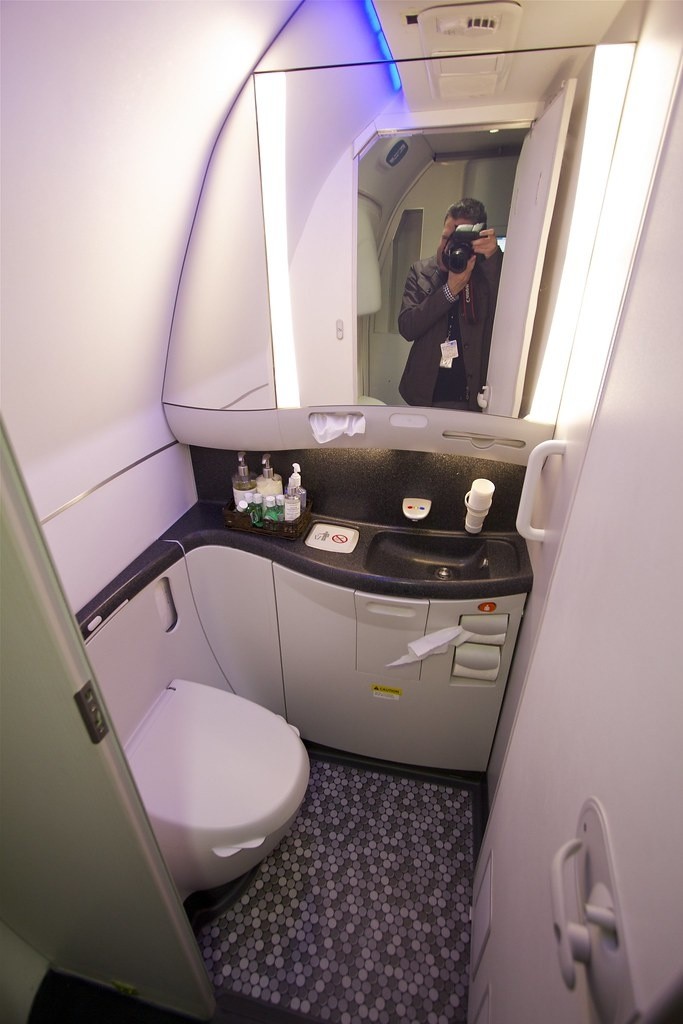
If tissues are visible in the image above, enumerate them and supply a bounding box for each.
[309,411,365,445]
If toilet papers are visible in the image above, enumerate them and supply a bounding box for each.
[386,613,510,682]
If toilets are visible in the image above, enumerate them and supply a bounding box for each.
[119,676,311,905]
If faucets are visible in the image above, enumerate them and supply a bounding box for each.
[401,497,433,523]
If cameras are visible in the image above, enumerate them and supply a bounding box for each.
[442,222,487,274]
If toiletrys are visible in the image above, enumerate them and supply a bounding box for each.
[231,451,308,529]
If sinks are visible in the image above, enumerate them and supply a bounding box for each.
[363,530,521,583]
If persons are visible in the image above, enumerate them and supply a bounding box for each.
[395,197,503,414]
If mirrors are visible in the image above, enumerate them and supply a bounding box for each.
[252,43,637,417]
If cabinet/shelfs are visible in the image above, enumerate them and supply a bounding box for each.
[273,562,525,774]
[62,540,237,751]
